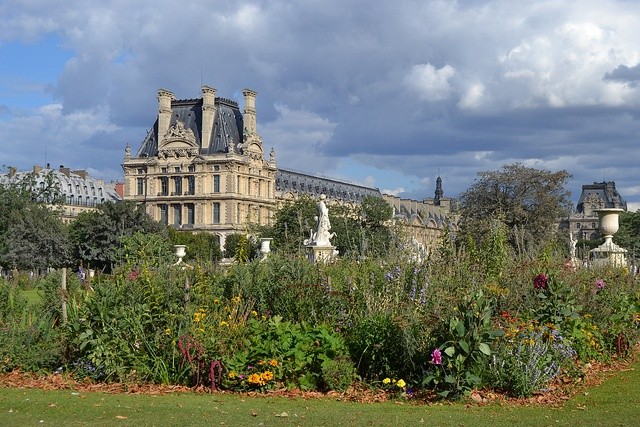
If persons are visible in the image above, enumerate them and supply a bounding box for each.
[312,193,332,246]
